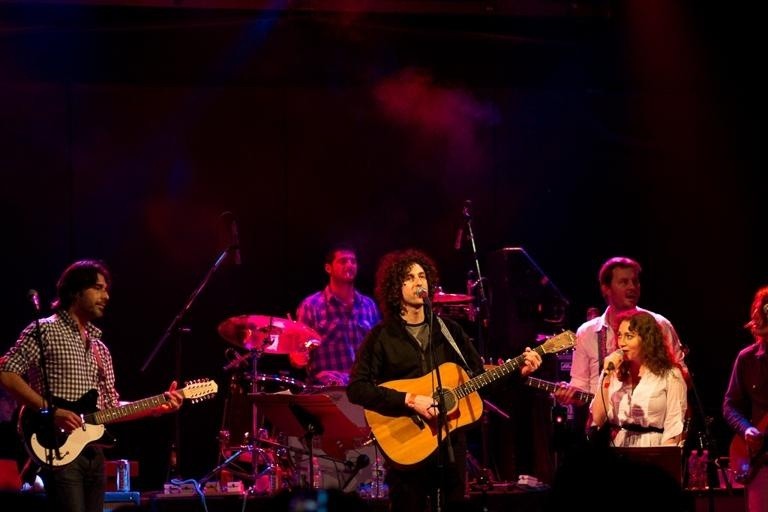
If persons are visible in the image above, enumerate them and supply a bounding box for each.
[586,312,688,455]
[345,246,544,512]
[722,284,768,512]
[286,246,379,378]
[0,391,44,511]
[470,241,568,484]
[0,259,184,512]
[553,255,695,407]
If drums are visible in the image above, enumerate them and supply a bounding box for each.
[221,372,306,462]
[269,385,386,491]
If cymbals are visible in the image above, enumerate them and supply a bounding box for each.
[219,315,322,353]
[432,292,473,302]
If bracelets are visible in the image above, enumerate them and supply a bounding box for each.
[407,393,418,407]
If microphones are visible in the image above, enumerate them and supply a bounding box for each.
[26,286,43,313]
[453,199,473,251]
[412,287,438,315]
[606,348,627,373]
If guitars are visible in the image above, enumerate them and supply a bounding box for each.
[728,414,768,490]
[25,378,219,471]
[479,353,691,440]
[364,329,576,465]
